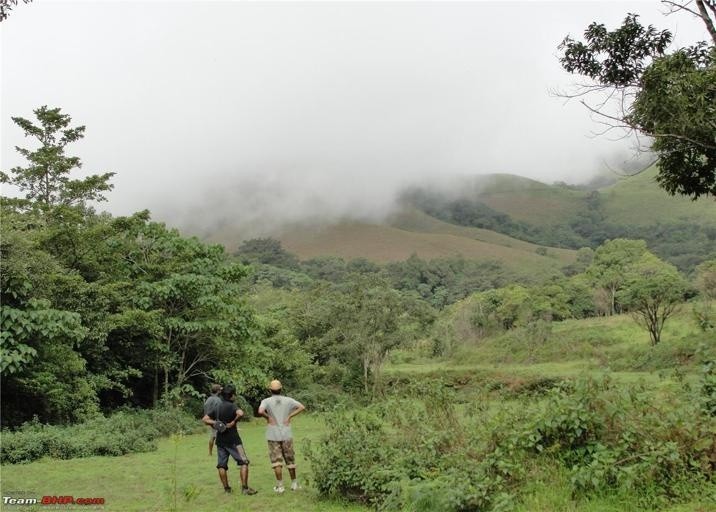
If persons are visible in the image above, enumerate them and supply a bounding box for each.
[201,384,257,495]
[258,379,305,493]
[203,384,222,455]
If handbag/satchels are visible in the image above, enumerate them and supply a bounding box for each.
[213,421,225,433]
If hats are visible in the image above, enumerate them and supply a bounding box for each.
[270,380,281,390]
[212,384,223,391]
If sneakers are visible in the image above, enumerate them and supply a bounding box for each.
[273,486,283,493]
[242,488,257,496]
[290,483,300,491]
